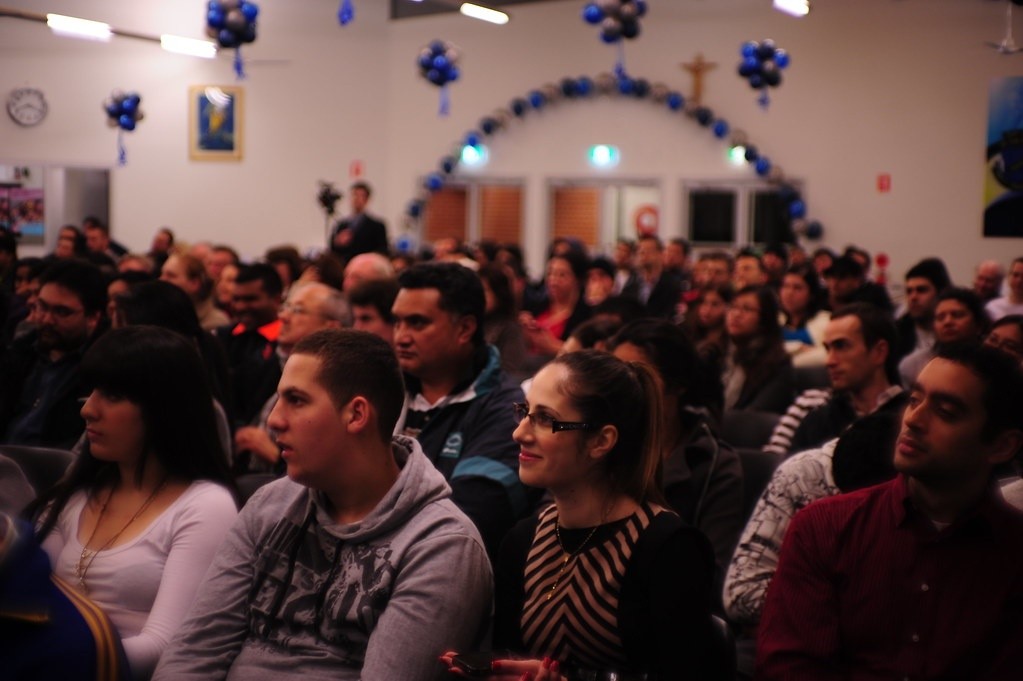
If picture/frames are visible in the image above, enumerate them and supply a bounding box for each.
[189,84,244,161]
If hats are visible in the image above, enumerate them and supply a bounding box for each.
[905,255,952,292]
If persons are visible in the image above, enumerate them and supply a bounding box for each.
[437,349,714,681]
[0,218,1023,681]
[755,342,1023,681]
[38,325,237,681]
[331,183,390,268]
[152,328,495,681]
[1,513,131,681]
[547,497,616,601]
[0,197,43,232]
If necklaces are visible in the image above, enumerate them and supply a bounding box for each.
[76,485,155,594]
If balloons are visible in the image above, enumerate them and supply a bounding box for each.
[103,90,144,131]
[208,1,259,47]
[738,39,790,88]
[415,39,458,85]
[584,0,648,43]
[404,72,824,244]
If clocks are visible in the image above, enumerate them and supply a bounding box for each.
[7,88,49,126]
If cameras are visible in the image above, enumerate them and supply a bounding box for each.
[319,189,341,208]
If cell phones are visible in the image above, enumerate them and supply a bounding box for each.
[453,649,514,675]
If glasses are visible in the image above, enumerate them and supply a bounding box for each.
[512,402,591,434]
